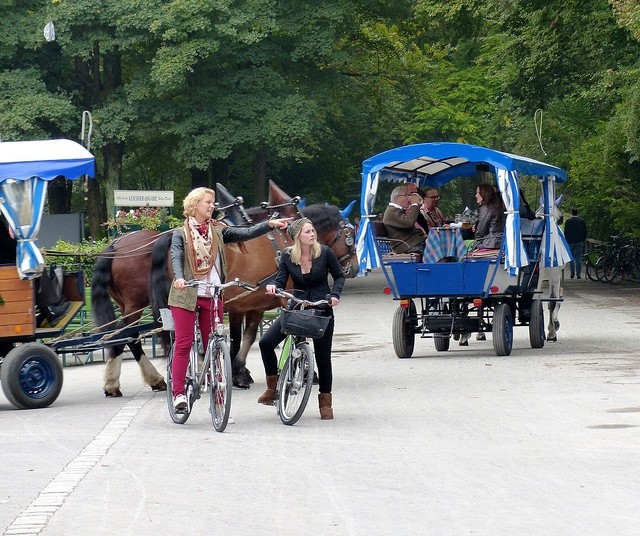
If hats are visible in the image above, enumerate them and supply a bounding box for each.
[286,219,312,242]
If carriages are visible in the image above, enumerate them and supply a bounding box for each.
[0,139,360,409]
[355,142,574,358]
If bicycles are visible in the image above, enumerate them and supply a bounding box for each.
[262,287,333,425]
[581,235,640,285]
[165,278,256,432]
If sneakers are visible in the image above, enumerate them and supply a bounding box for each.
[173,393,188,414]
[208,404,235,424]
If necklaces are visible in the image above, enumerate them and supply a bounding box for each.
[301,260,312,266]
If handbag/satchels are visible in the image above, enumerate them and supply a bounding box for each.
[283,300,325,336]
[45,261,69,304]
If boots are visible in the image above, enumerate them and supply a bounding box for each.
[318,393,334,420]
[259,374,280,403]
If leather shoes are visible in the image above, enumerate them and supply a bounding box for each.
[43,301,72,328]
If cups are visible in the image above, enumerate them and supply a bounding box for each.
[409,193,423,205]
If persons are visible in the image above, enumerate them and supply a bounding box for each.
[403,182,427,235]
[345,217,354,228]
[354,218,360,234]
[421,188,445,230]
[378,212,384,222]
[258,217,345,420]
[464,183,508,249]
[415,187,429,235]
[0,219,72,328]
[564,208,587,279]
[382,185,452,263]
[168,186,295,408]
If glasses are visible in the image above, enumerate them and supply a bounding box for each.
[426,195,440,200]
[397,194,410,198]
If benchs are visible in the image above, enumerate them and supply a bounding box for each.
[373,219,420,261]
[461,233,504,259]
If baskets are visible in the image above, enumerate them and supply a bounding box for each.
[279,305,331,339]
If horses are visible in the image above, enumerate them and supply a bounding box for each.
[89,200,360,398]
[534,192,568,341]
[453,327,486,346]
